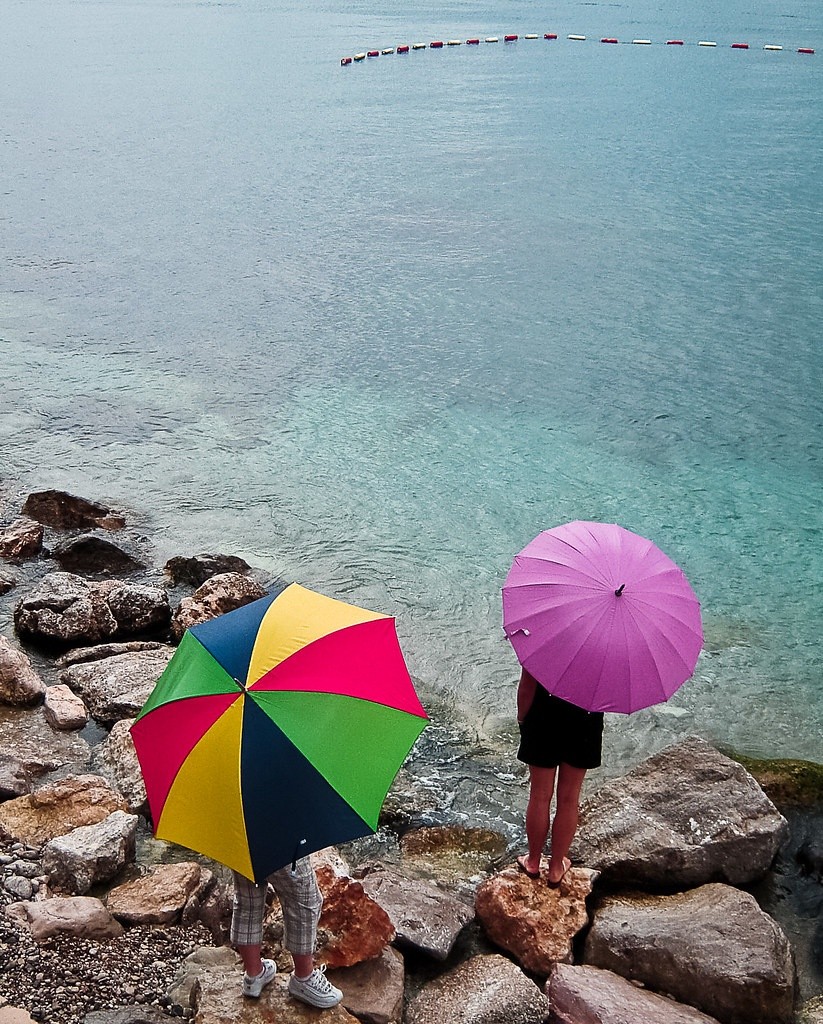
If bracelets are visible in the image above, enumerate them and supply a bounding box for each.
[517,716,523,723]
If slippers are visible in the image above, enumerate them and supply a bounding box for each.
[517,854,540,880]
[547,857,570,889]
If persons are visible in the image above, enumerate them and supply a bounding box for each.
[516,666,604,889]
[229,856,344,1009]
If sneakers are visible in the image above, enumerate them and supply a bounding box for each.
[287,963,343,1008]
[243,957,276,996]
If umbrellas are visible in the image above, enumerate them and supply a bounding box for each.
[501,520,704,716]
[130,581,430,887]
